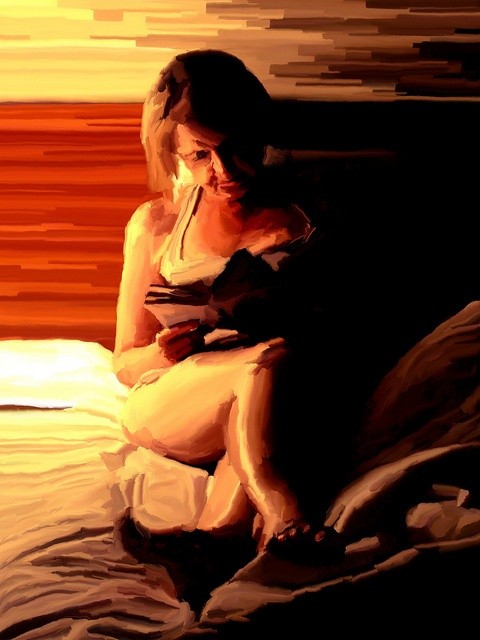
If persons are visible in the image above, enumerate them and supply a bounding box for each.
[112,47,350,561]
